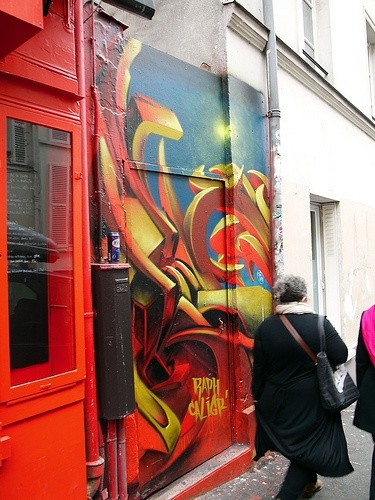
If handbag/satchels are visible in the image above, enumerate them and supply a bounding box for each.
[317,316,362,413]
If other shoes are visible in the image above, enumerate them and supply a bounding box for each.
[301,479,322,498]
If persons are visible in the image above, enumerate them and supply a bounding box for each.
[251,275,354,499]
[352,304,375,500]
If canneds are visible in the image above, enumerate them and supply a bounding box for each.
[107,232,120,264]
